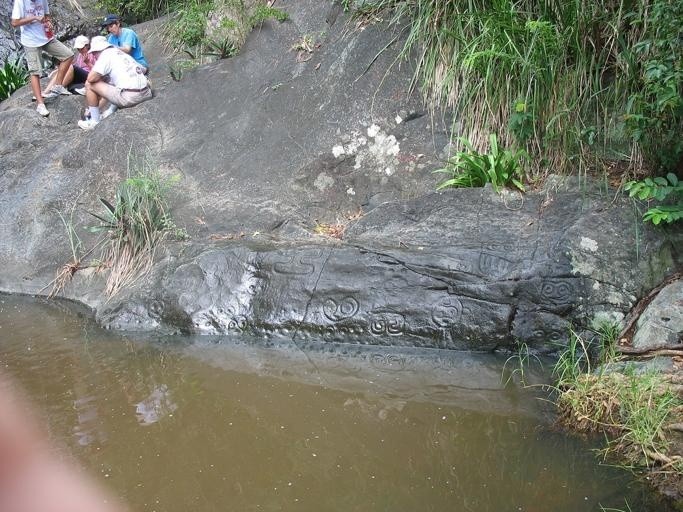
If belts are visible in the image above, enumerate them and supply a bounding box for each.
[121,87,148,92]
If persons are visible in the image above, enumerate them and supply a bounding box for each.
[76,35,152,132]
[30,35,96,100]
[73,14,149,97]
[10,0,74,117]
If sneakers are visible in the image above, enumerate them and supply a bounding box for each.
[32,84,116,130]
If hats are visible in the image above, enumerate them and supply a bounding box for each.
[87,35,114,53]
[74,35,90,49]
[102,14,119,26]
